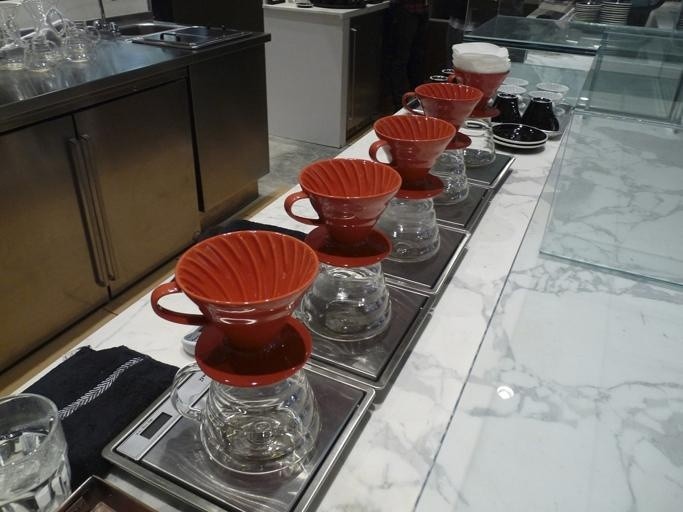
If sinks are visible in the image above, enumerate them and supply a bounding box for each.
[117,23,177,35]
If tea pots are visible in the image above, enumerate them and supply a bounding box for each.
[54,19,101,64]
[376,198,440,263]
[428,149,468,206]
[167,363,322,473]
[457,119,495,167]
[17,32,61,73]
[300,261,392,343]
[0,0,25,71]
[22,0,69,60]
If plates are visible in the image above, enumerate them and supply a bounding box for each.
[194,316,313,386]
[490,122,548,149]
[303,224,393,267]
[443,131,471,150]
[390,175,444,200]
[465,106,501,118]
[571,1,633,26]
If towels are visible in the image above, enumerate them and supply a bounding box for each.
[0,344,184,494]
[219,217,309,245]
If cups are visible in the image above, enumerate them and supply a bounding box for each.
[0,393,71,512]
[518,96,560,131]
[489,93,521,123]
[284,156,404,245]
[367,112,458,182]
[401,81,485,133]
[144,228,322,349]
[446,66,510,110]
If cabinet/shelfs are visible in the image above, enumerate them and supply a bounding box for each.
[187,33,269,230]
[262,3,391,149]
[1,67,202,368]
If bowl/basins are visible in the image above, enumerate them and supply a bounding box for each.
[498,76,529,86]
[535,82,569,94]
[527,89,563,104]
[493,83,527,96]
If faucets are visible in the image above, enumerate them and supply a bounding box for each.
[98,1,108,30]
